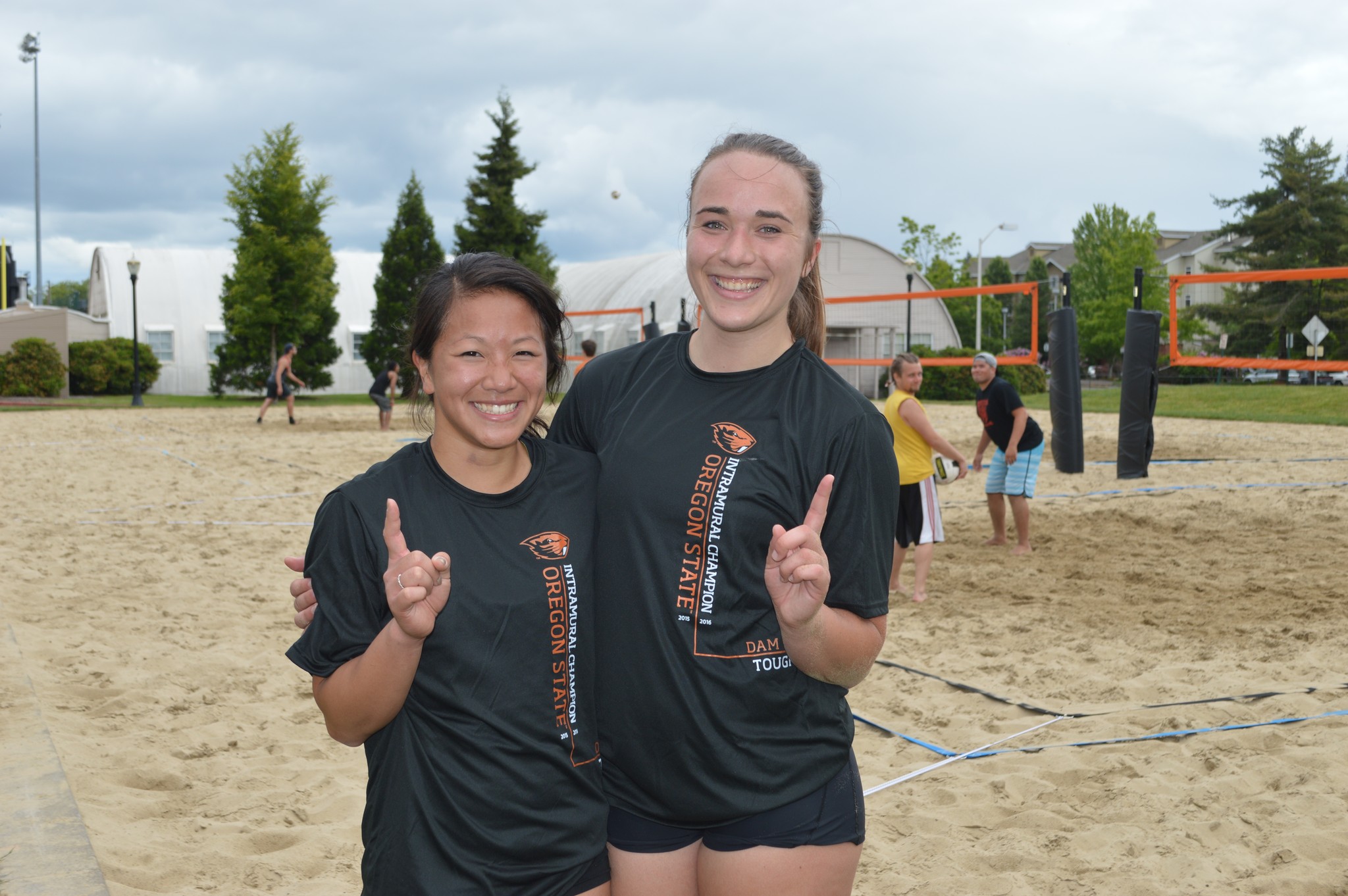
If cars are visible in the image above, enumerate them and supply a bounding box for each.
[1287,369,1348,386]
[1242,368,1302,387]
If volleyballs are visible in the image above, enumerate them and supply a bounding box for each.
[611,191,621,200]
[931,453,960,486]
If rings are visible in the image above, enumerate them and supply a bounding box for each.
[398,574,405,589]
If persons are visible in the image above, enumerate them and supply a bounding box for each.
[971,352,1043,553]
[573,339,597,377]
[286,252,601,894]
[882,353,968,605]
[369,363,400,431]
[283,132,900,896]
[255,344,306,424]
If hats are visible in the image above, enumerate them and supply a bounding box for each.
[974,352,998,370]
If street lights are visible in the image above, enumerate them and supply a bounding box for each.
[904,257,916,354]
[1001,302,1009,351]
[20,29,43,308]
[126,252,147,407]
[975,221,1020,349]
